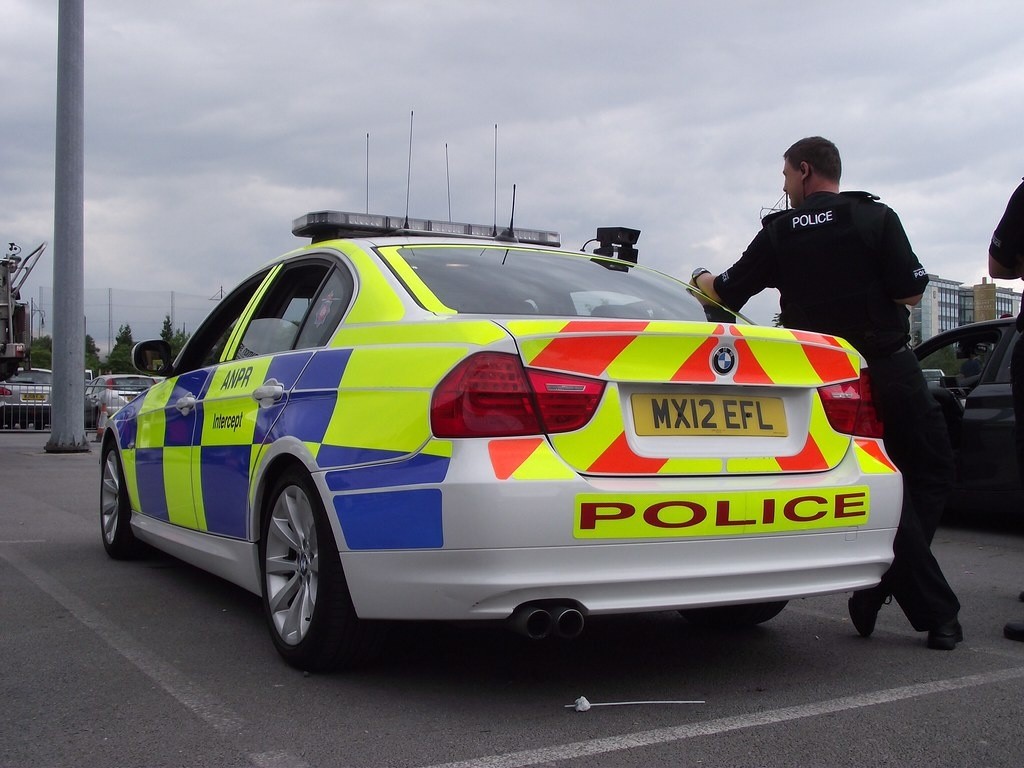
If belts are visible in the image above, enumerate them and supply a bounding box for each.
[892,343,911,355]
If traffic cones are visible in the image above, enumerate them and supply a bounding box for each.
[91,404,107,444]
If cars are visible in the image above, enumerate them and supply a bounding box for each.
[83,374,159,428]
[103,214,907,671]
[886,315,1024,519]
[0,368,54,425]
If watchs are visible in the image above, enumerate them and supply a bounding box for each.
[692,268,711,287]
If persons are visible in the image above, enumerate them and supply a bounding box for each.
[686,288,736,324]
[688,136,962,650]
[990,178,1023,641]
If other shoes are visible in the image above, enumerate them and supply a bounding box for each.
[1003,623,1024,642]
[926,620,963,652]
[848,578,893,636]
[1019,591,1024,603]
